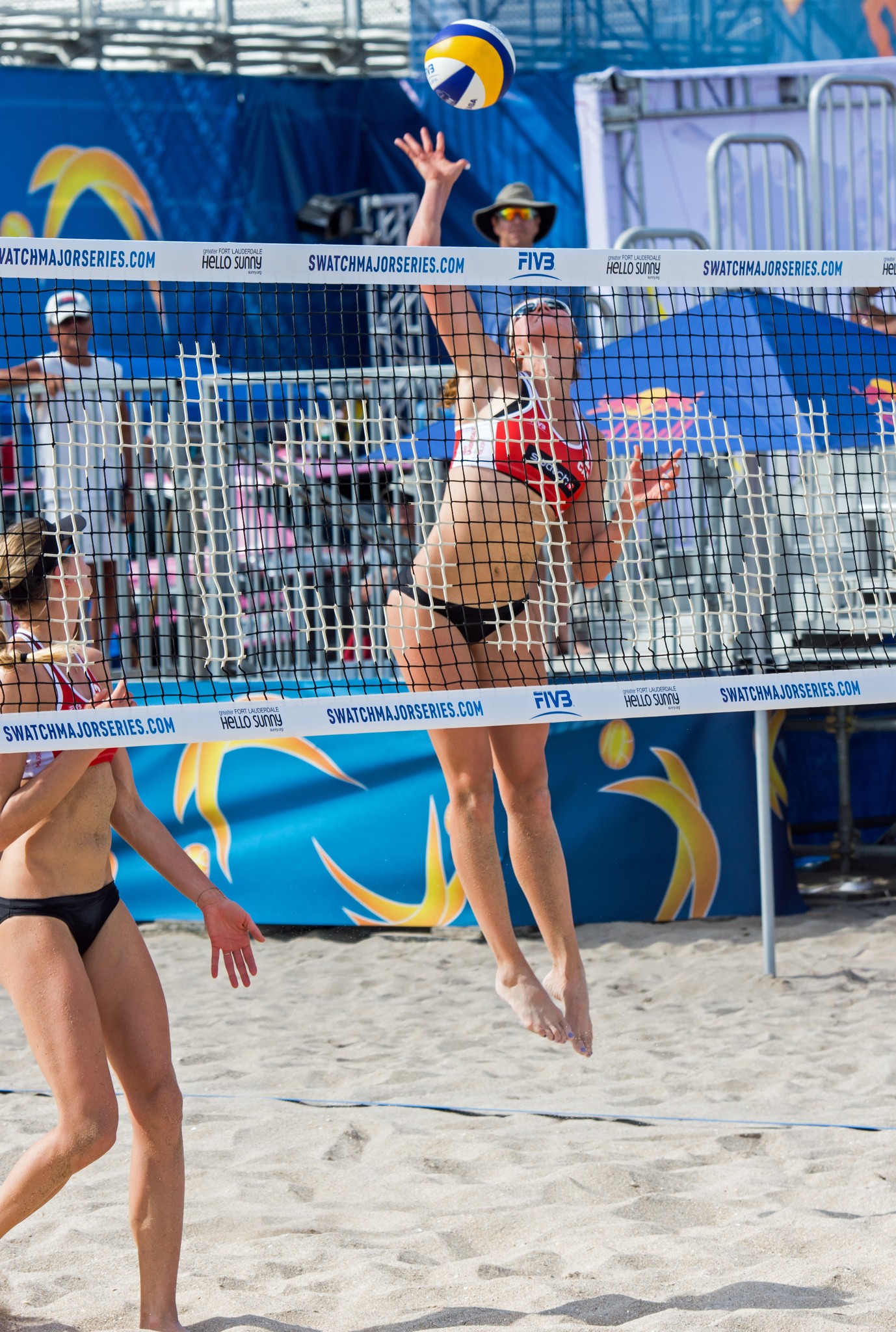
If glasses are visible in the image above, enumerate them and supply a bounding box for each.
[499,207,538,221]
[509,299,575,351]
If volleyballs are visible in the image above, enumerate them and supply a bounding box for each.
[424,18,517,111]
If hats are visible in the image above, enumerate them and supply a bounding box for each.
[473,183,557,244]
[1,515,87,607]
[45,290,88,325]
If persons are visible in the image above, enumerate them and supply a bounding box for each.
[352,474,422,606]
[459,182,597,363]
[0,291,142,669]
[0,517,265,1332]
[388,131,683,1057]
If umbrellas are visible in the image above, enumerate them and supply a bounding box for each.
[356,288,896,983]
